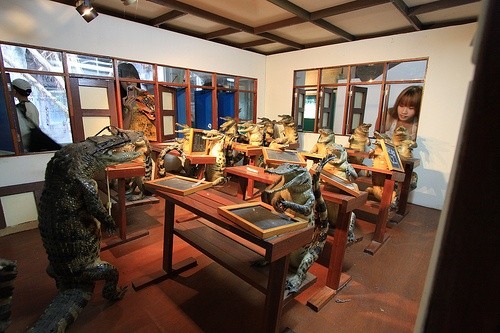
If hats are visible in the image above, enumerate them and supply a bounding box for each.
[11,79,32,96]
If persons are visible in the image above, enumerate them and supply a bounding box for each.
[114,63,141,130]
[374,85,422,139]
[11,78,40,152]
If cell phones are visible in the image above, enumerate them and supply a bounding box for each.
[127,86,135,99]
